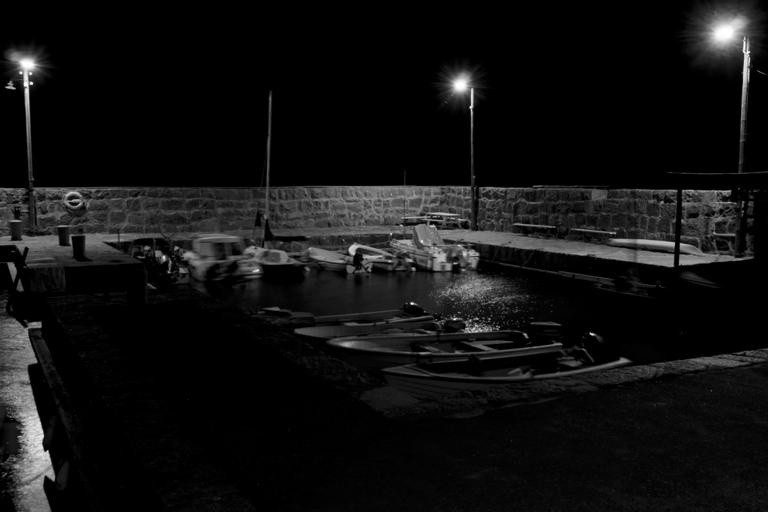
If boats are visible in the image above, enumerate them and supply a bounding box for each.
[179,233,265,281]
[348,240,417,273]
[390,221,481,273]
[379,331,633,400]
[305,245,365,273]
[128,236,192,287]
[323,319,564,374]
[279,301,434,327]
[291,314,467,349]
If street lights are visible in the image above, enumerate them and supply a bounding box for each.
[6,54,43,236]
[712,22,753,258]
[449,78,480,231]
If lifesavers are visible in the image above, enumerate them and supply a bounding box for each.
[63,190,84,209]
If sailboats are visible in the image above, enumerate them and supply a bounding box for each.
[239,89,309,275]
